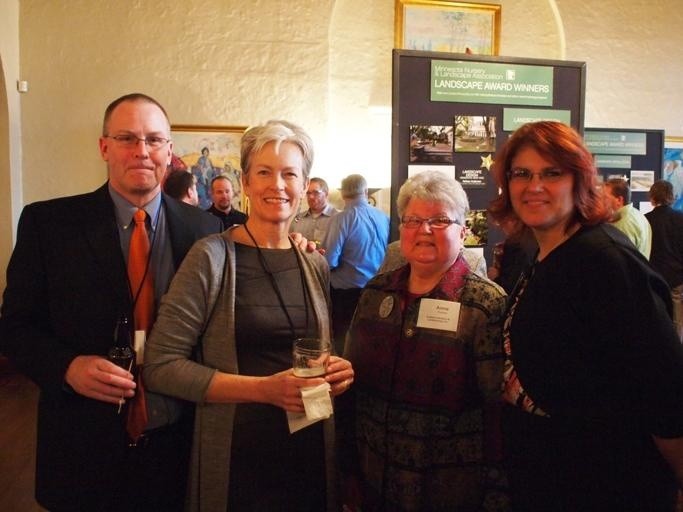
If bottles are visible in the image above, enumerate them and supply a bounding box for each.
[97,313,137,418]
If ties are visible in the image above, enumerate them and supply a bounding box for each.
[123,210,157,442]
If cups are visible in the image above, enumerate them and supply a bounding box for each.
[292,338,332,378]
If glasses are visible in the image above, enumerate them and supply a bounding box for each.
[399,213,464,229]
[107,134,171,148]
[507,166,572,182]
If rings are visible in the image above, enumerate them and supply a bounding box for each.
[340,380,349,391]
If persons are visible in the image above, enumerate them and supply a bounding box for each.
[0,92,225,512]
[291,174,390,358]
[162,165,248,230]
[482,120,680,512]
[641,178,683,346]
[334,169,511,511]
[194,147,213,188]
[139,118,356,509]
[600,177,653,263]
[484,222,529,293]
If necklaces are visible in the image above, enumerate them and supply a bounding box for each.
[241,220,309,341]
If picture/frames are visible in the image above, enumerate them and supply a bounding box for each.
[166,125,250,232]
[394,0,502,62]
